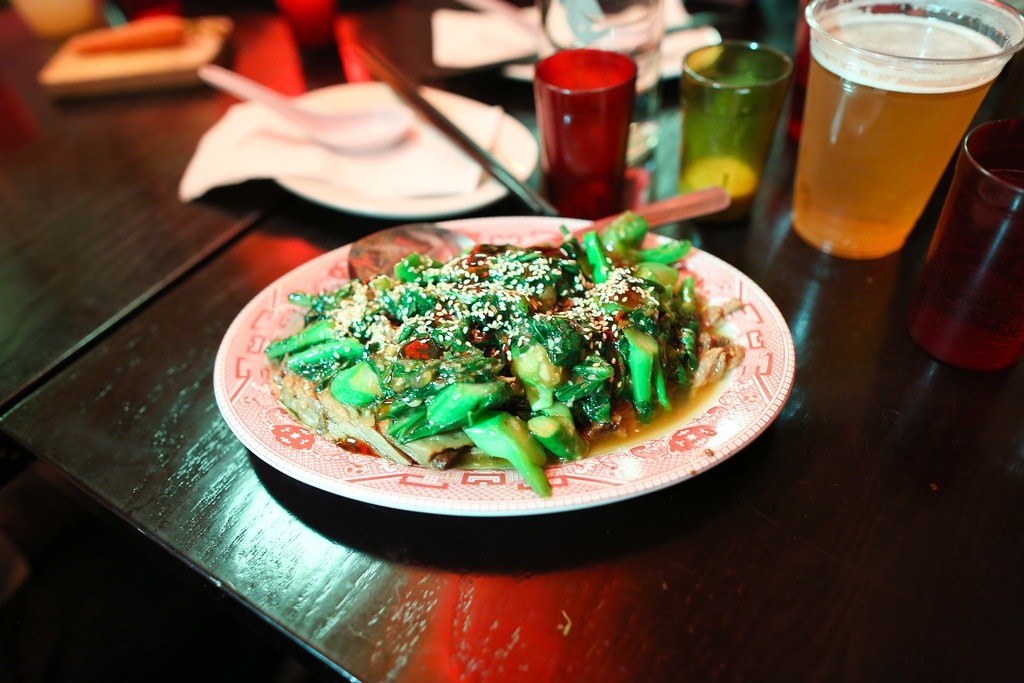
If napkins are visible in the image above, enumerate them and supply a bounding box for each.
[431,0,690,70]
[178,105,504,203]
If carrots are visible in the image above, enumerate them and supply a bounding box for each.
[72,14,183,52]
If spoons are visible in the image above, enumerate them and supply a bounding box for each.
[348,186,730,283]
[196,63,409,154]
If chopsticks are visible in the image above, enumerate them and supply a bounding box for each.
[354,44,558,216]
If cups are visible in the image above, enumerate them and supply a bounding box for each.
[675,41,794,223]
[909,111,1024,372]
[567,18,659,168]
[792,0,1024,260]
[533,48,638,223]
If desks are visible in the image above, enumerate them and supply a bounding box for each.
[0,0,1024,683]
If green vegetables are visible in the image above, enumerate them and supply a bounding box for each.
[264,211,698,498]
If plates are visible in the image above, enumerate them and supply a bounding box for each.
[498,6,723,83]
[212,216,795,517]
[177,80,540,221]
